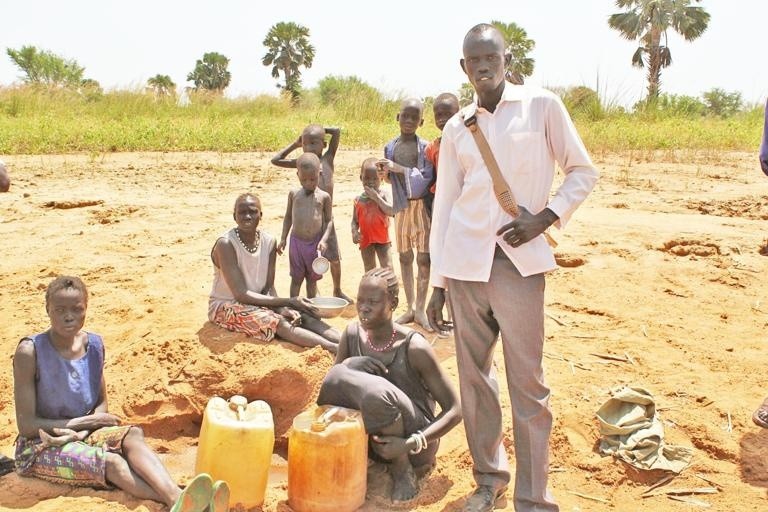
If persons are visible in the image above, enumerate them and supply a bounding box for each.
[11,272,232,512]
[274,150,336,301]
[314,267,465,500]
[268,122,359,304]
[373,97,438,334]
[343,156,398,273]
[424,91,461,222]
[206,191,342,352]
[426,22,603,511]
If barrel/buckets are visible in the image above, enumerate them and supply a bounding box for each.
[195,392,276,510]
[288,404,369,512]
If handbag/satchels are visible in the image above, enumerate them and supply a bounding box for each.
[462,106,558,248]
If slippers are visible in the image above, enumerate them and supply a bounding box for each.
[752,398,768,429]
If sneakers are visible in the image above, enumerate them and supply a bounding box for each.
[209,480,230,512]
[462,481,508,512]
[169,473,212,511]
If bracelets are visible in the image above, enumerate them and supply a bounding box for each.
[409,430,430,455]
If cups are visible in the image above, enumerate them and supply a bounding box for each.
[310,249,330,281]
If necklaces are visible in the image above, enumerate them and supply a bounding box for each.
[236,229,260,255]
[365,330,398,352]
